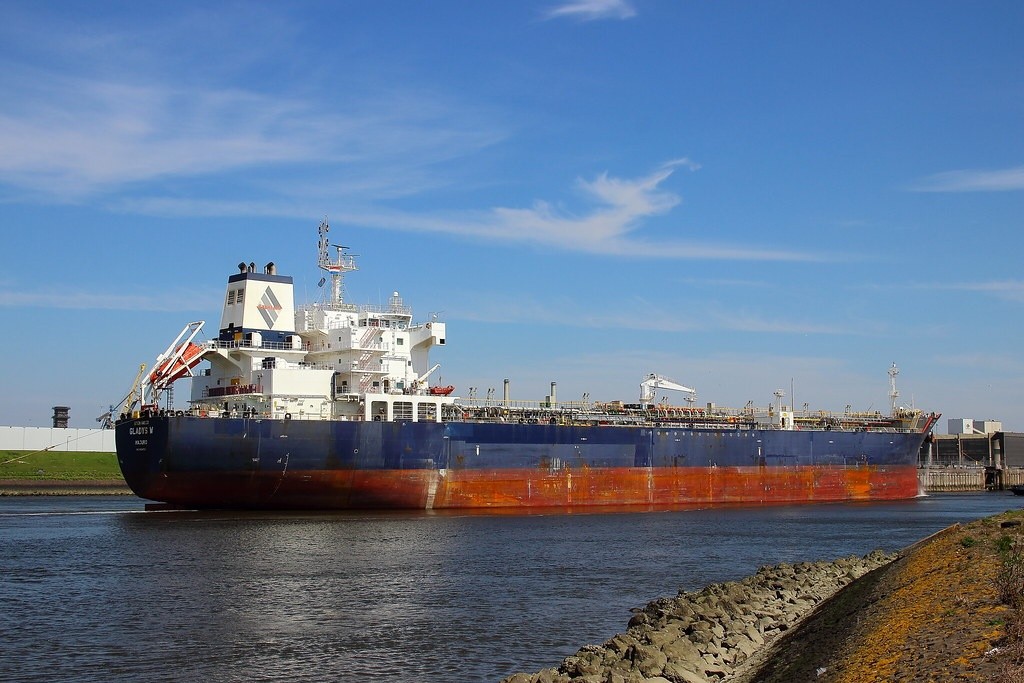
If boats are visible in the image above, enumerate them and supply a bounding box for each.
[430,384,455,394]
[149,341,205,389]
[100,213,947,521]
